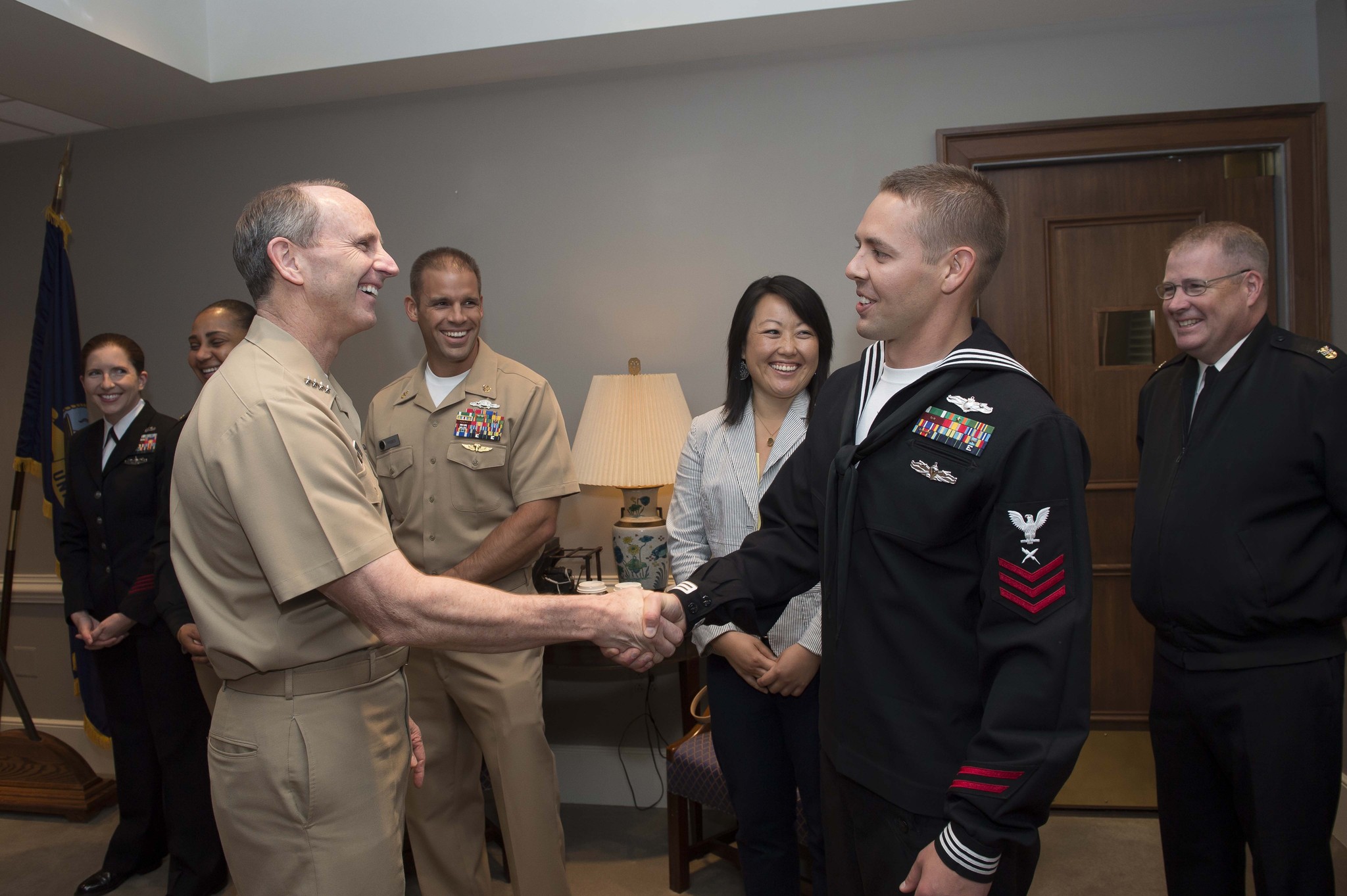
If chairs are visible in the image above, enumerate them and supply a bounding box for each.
[664,685,731,892]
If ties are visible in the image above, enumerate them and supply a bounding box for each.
[1189,367,1220,431]
[102,428,118,473]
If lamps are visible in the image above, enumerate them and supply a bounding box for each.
[572,373,691,592]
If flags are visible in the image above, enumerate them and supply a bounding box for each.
[13,204,107,750]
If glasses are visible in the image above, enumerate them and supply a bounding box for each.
[1154,268,1253,300]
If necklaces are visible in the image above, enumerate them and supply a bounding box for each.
[751,406,783,446]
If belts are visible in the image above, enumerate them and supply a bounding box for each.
[226,641,409,697]
[489,568,528,591]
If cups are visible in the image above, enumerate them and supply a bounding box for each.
[577,581,607,595]
[614,582,643,592]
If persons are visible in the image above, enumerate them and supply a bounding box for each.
[1131,226,1347,896]
[167,180,687,895]
[600,163,1097,896]
[666,275,842,896]
[362,248,581,896]
[159,299,256,718]
[64,331,229,896]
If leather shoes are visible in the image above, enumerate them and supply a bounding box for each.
[73,859,163,896]
